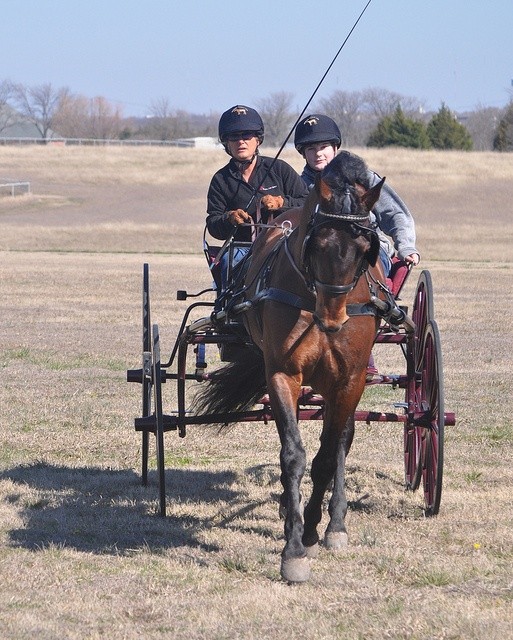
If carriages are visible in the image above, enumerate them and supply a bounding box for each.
[128,154,455,583]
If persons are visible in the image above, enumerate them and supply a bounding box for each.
[206,105,309,293]
[293,114,420,281]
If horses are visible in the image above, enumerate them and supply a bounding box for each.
[190,150,389,583]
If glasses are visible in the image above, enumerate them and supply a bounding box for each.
[223,134,258,141]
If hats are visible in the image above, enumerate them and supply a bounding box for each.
[295,115,341,150]
[219,106,264,132]
